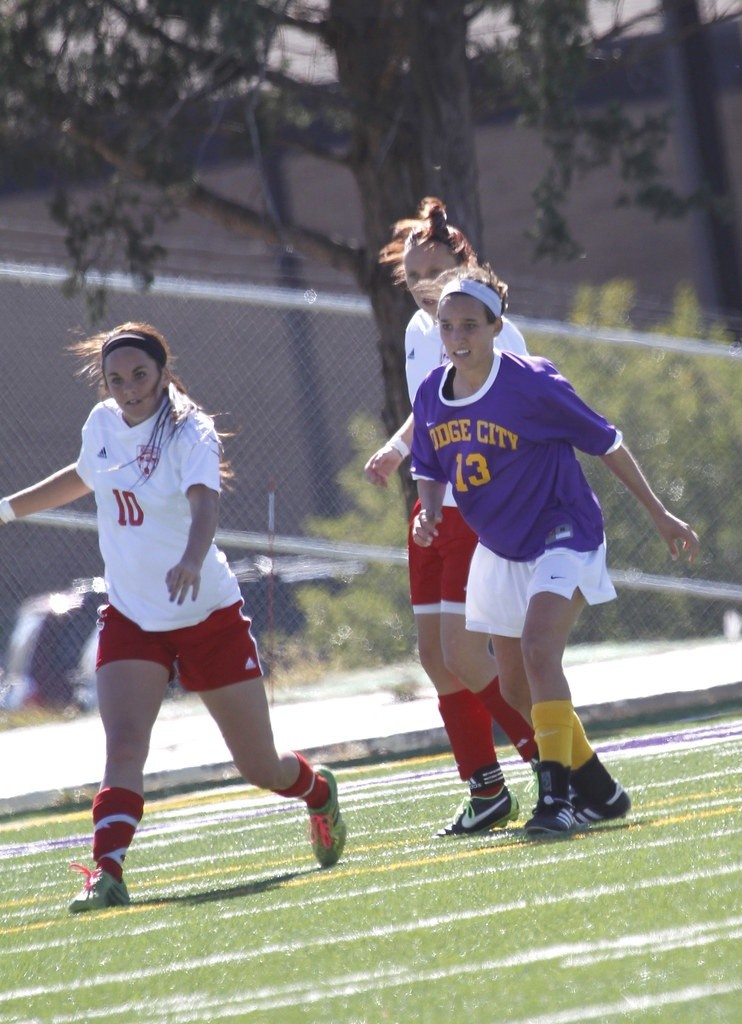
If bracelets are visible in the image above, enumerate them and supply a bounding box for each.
[0,497,16,524]
[385,438,411,459]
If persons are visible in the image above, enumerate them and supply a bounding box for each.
[411,267,700,835]
[0,322,346,913]
[366,197,542,839]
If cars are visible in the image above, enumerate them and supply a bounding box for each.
[15,557,358,716]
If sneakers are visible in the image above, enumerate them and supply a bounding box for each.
[570,777,630,828]
[305,764,346,866]
[524,795,576,835]
[67,864,130,914]
[436,785,520,841]
[525,769,540,804]
